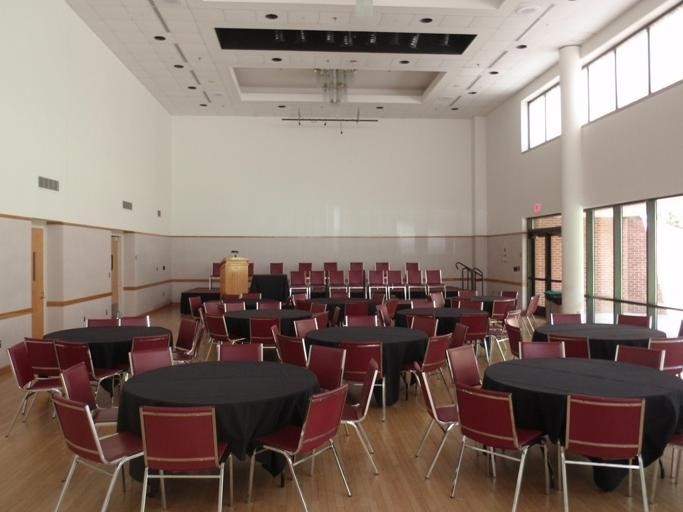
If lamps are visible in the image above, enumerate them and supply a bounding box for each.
[314,68,355,105]
[268,27,449,49]
[281,107,378,134]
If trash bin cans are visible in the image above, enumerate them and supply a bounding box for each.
[545,290,562,323]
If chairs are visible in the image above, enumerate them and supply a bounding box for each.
[414,361,461,479]
[190,261,542,347]
[649,431,683,503]
[62,363,130,494]
[558,393,649,511]
[217,343,264,362]
[6,315,201,438]
[304,344,349,437]
[445,345,485,457]
[205,315,232,361]
[139,406,234,512]
[450,384,551,512]
[51,393,144,512]
[485,296,682,376]
[400,334,452,400]
[276,334,307,365]
[247,384,351,512]
[309,358,379,477]
[340,341,387,422]
